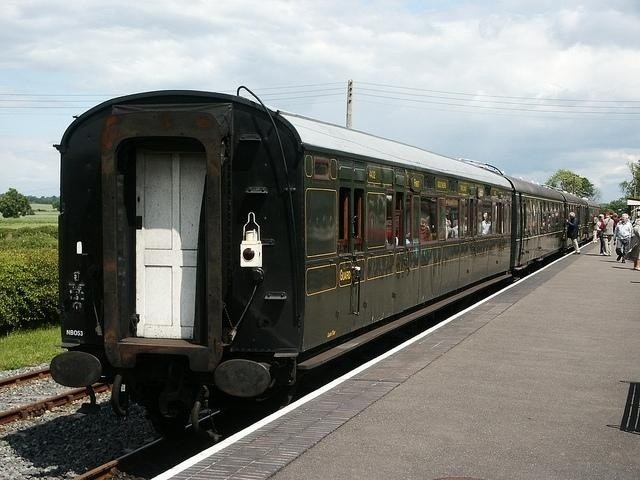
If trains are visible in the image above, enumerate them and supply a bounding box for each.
[50,86,599,435]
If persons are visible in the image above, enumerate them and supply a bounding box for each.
[419,215,433,241]
[591,209,640,271]
[565,211,581,255]
[481,211,491,235]
[446,217,468,240]
